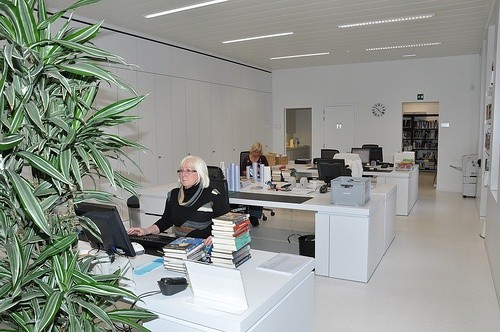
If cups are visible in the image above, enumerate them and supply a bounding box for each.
[288,177,296,188]
[300,177,307,187]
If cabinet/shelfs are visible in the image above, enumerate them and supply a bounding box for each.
[402,119,438,172]
[476,5,500,302]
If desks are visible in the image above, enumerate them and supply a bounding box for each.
[72,240,314,332]
[137,164,419,284]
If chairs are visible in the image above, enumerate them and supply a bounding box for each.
[240,151,275,221]
[207,166,230,213]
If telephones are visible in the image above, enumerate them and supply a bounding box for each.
[157,276,188,295]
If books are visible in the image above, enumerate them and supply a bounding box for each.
[486,104,492,119]
[484,159,489,186]
[162,237,206,274]
[210,213,252,268]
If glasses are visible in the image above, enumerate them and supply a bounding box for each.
[177,169,196,172]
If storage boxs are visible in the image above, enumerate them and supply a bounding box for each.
[330,176,370,207]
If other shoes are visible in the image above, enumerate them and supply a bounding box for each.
[250,216,259,227]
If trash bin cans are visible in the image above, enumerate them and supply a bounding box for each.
[298,234,315,271]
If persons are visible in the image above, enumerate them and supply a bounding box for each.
[126,155,227,246]
[242,142,269,227]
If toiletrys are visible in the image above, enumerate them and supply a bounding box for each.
[290,137,298,146]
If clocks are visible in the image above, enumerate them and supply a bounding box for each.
[372,103,385,117]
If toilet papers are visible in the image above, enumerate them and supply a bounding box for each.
[264,166,270,183]
